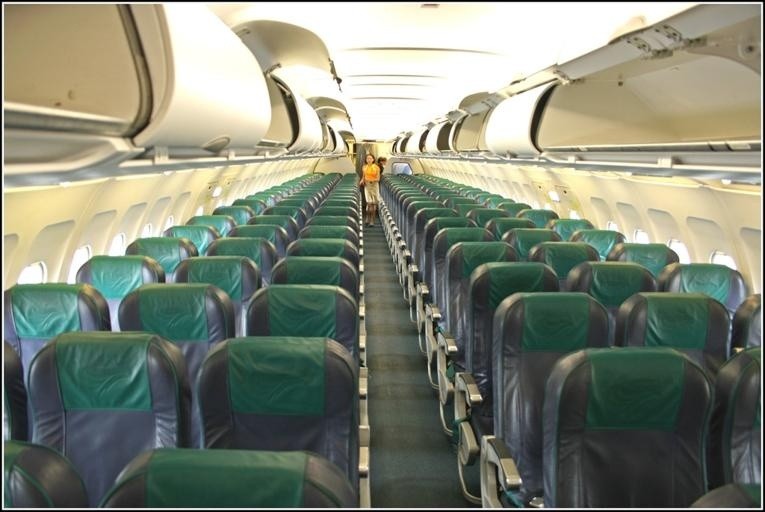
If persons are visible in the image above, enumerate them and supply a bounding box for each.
[358,153,381,227]
[377,157,387,173]
